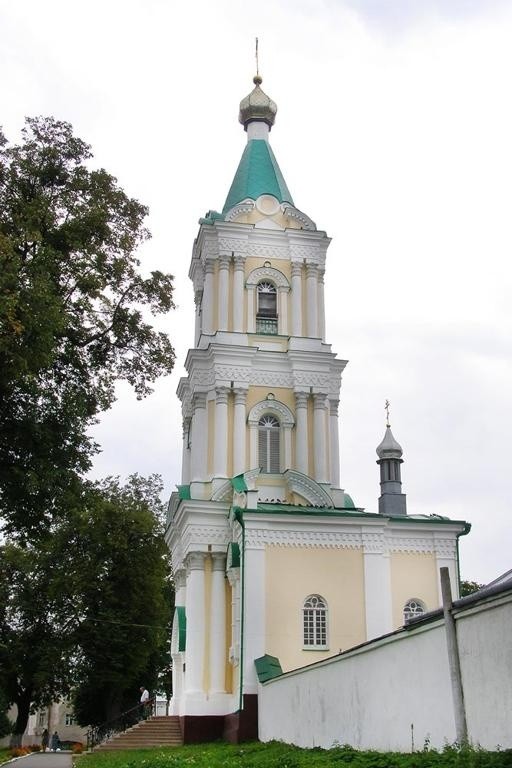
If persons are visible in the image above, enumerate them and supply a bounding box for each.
[140,686,149,720]
[52,731,58,752]
[41,729,49,753]
